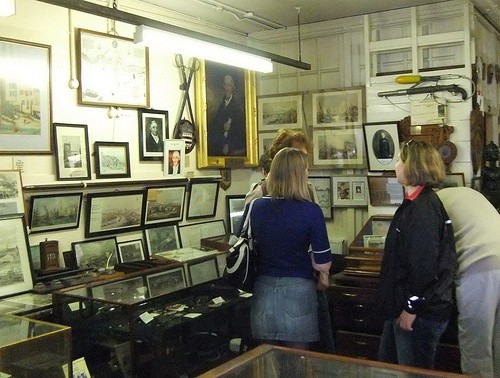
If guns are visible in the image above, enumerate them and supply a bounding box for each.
[377,75,467,102]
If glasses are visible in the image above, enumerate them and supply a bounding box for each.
[405,139,413,149]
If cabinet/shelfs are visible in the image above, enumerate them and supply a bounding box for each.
[196,282,471,378]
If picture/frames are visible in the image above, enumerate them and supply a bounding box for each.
[0,29,466,336]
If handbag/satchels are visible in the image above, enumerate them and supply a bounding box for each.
[221,199,255,293]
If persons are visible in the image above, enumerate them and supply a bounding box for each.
[146,121,163,153]
[376,139,458,378]
[435,186,500,378]
[170,150,181,174]
[229,129,332,347]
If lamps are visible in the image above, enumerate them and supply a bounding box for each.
[133,24,273,74]
